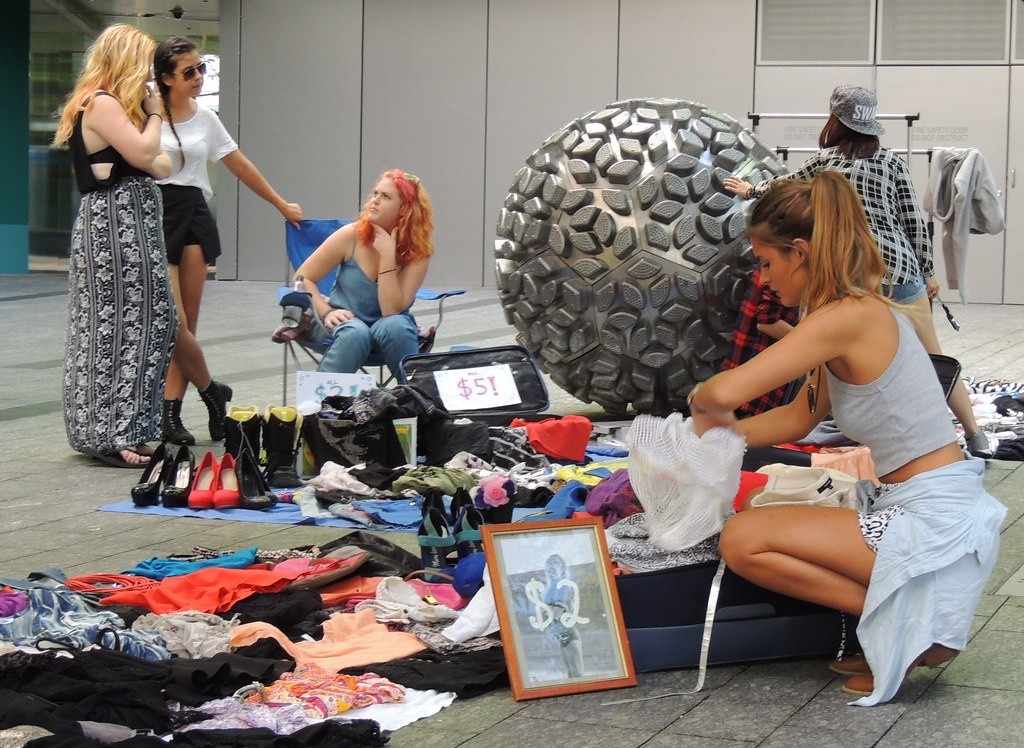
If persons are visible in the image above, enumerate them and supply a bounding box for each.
[292,168,436,385]
[724,84,990,452]
[541,554,584,678]
[689,170,1008,707]
[53,23,180,469]
[153,36,303,445]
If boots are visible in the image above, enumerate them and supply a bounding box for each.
[198,379,232,441]
[225,405,260,465]
[261,405,304,488]
[162,399,195,446]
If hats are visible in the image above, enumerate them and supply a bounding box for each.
[830,83,886,135]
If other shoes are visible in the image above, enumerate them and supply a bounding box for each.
[829,655,872,676]
[842,643,960,695]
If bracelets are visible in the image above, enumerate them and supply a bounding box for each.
[379,269,397,274]
[687,381,703,404]
[148,113,162,120]
[746,188,751,199]
[322,308,336,325]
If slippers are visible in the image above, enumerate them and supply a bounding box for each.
[98,442,155,468]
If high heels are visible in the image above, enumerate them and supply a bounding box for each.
[417,487,488,575]
[131,443,278,511]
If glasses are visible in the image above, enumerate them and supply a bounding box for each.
[403,173,420,185]
[163,63,206,81]
[807,364,821,413]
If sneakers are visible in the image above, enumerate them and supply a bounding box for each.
[964,432,992,459]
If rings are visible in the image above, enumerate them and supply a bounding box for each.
[933,291,936,293]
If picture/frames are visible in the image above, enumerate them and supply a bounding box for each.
[481,516,638,701]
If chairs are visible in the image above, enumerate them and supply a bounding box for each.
[283,219,467,407]
